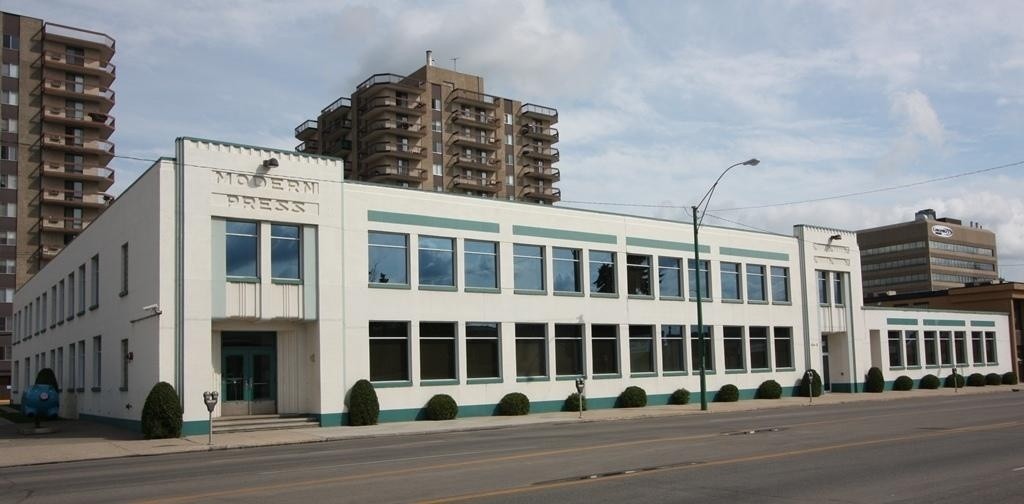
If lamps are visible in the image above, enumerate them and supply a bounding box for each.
[263,158,279,168]
[830,234,842,240]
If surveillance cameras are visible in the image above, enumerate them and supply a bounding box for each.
[142,303,158,312]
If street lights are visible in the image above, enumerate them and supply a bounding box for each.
[691,158,760,410]
